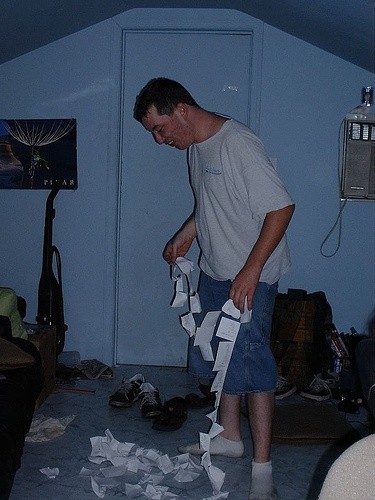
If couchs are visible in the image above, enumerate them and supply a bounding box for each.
[0,314,45,500]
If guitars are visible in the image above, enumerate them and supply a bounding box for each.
[35,177,68,360]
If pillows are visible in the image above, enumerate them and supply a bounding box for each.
[0,338,35,371]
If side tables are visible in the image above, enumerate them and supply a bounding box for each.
[29,325,57,409]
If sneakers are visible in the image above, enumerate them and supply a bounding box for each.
[300,371,336,401]
[274,375,297,399]
[109,373,146,407]
[139,382,162,416]
[152,397,188,431]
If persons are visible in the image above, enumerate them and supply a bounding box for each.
[133,77,296,500]
